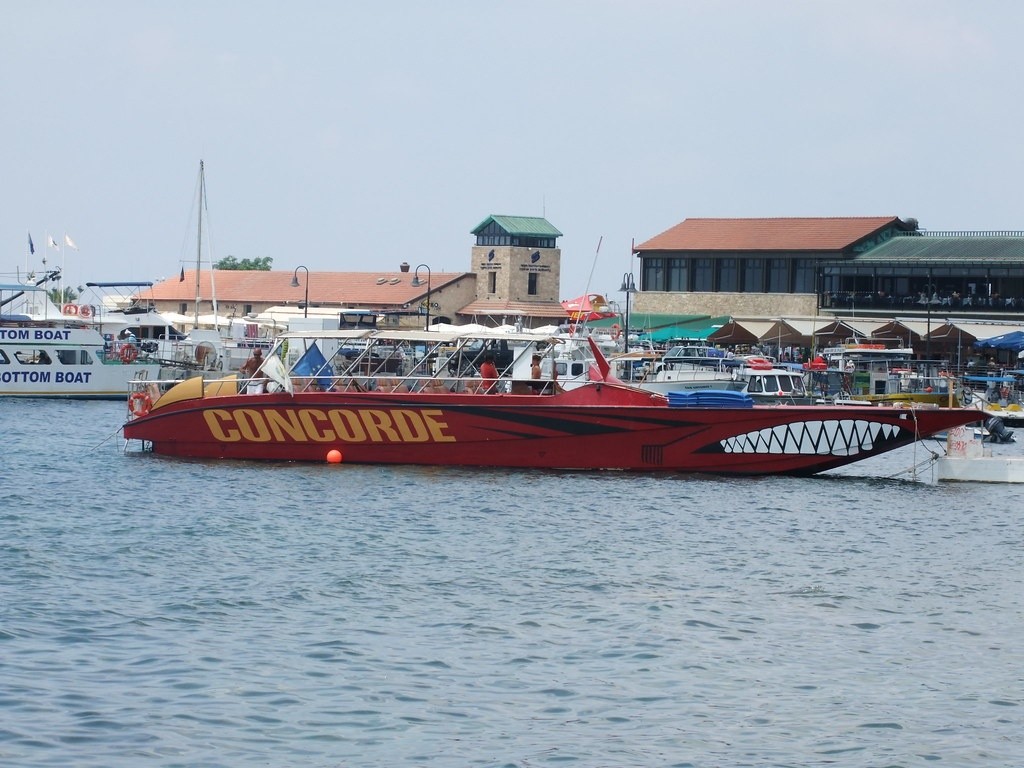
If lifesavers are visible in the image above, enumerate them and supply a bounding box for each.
[128,393,152,417]
[79,304,92,318]
[609,323,621,339]
[120,344,137,362]
[64,303,78,315]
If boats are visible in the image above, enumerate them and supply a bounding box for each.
[0,230,274,400]
[121,331,1024,477]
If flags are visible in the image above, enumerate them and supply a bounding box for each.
[64,232,76,248]
[29,233,34,255]
[260,338,293,398]
[292,343,334,391]
[47,233,57,248]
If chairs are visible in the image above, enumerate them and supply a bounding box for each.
[291,379,486,394]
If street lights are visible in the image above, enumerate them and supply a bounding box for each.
[617,272,638,379]
[291,266,308,318]
[917,291,943,389]
[411,263,432,331]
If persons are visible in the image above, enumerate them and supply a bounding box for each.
[532,355,541,395]
[569,322,575,338]
[938,355,1024,393]
[727,345,811,363]
[239,347,269,394]
[481,350,500,394]
[117,329,139,364]
[828,287,1001,309]
[553,361,559,395]
[38,353,48,363]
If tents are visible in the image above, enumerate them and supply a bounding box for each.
[973,331,1024,366]
